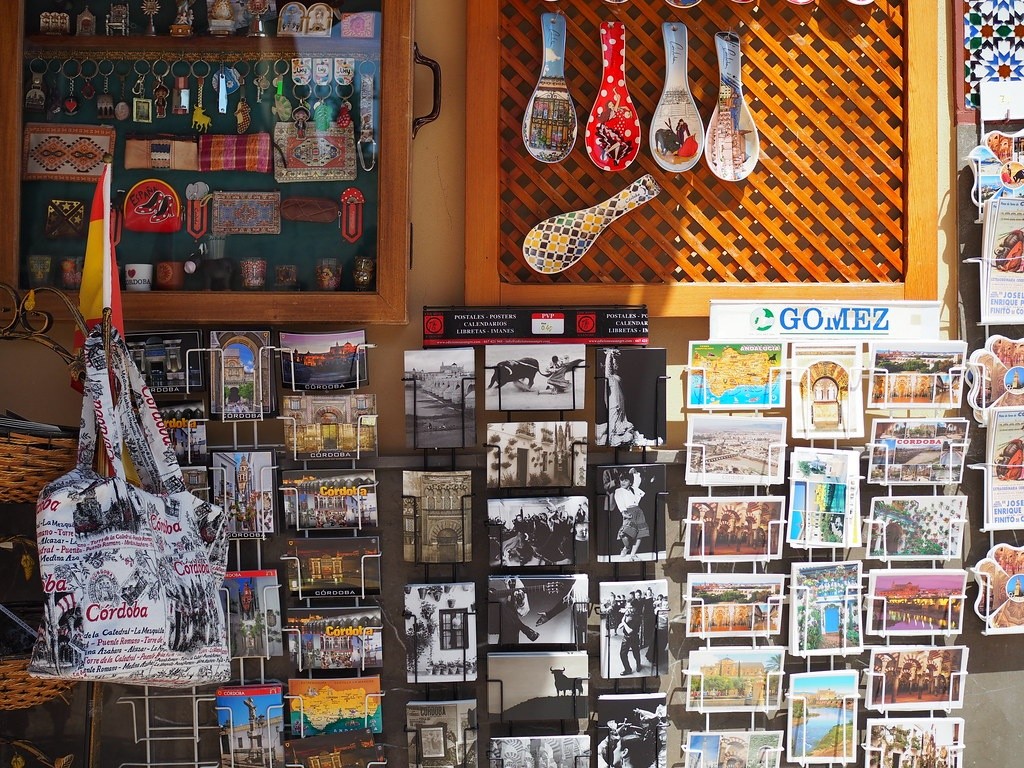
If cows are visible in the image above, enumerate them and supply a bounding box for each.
[549,667,583,697]
[488,357,551,390]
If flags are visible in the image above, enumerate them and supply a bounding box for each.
[69,162,141,488]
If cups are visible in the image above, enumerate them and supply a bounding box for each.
[203,258,235,290]
[61,255,83,290]
[28,254,54,288]
[124,264,153,290]
[240,257,266,290]
[316,258,342,290]
[273,265,297,290]
[157,262,183,290]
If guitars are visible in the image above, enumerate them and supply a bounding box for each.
[489,579,564,604]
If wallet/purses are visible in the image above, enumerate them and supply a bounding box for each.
[200,190,281,235]
[123,132,199,170]
[273,122,359,184]
[198,130,273,176]
[22,121,116,182]
[280,198,342,229]
[109,178,185,247]
[42,196,88,240]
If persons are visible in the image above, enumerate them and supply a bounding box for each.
[604,349,634,446]
[503,498,587,566]
[603,467,650,561]
[488,576,588,644]
[537,354,571,395]
[600,586,669,676]
[598,708,667,768]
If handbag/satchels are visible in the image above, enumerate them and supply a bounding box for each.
[25,325,233,689]
